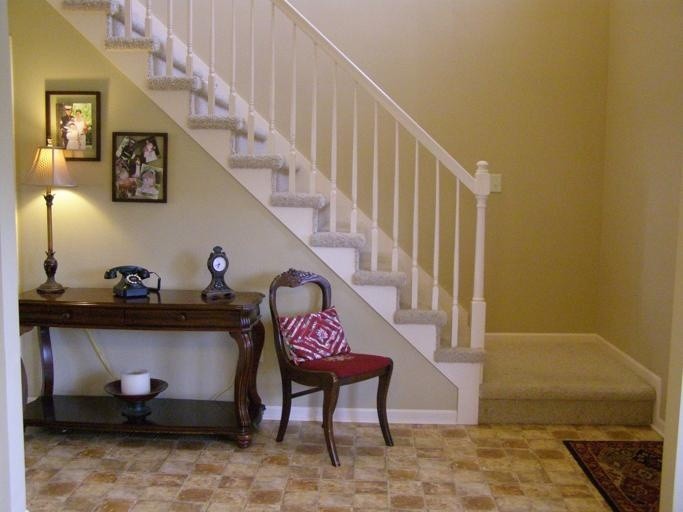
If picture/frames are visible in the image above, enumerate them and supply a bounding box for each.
[43,88,168,203]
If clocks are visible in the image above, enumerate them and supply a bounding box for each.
[200,245,235,300]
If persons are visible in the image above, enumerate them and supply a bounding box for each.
[112,134,164,200]
[73,109,86,149]
[63,120,78,150]
[59,106,73,150]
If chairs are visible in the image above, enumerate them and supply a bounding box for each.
[269,268,394,467]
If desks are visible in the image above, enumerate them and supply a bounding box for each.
[18,286,268,449]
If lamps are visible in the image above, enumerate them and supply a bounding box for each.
[21,136,79,294]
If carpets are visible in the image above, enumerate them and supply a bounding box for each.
[561,440,663,512]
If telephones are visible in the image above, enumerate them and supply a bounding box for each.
[104,265,150,298]
